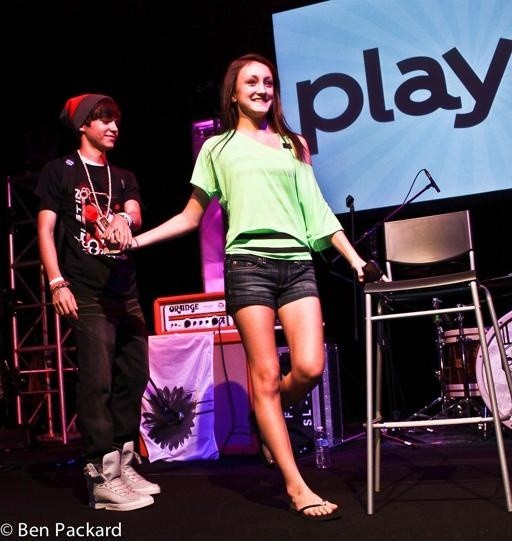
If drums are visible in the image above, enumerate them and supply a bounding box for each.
[440,327,490,398]
[475,311,512,429]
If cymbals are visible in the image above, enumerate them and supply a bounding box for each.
[468,273,512,287]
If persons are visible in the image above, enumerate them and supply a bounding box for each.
[100,55,387,521]
[36,93,160,512]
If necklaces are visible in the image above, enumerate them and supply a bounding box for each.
[77,150,112,232]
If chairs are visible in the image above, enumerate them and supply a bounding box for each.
[364,208,512,513]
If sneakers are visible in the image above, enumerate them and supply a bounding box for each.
[119,440,161,494]
[83,450,154,510]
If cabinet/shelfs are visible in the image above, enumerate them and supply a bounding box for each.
[133,330,262,464]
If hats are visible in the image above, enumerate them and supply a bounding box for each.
[59,93,113,130]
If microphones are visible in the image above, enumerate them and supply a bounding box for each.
[423,168,442,195]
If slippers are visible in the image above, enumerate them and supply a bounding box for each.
[288,498,342,521]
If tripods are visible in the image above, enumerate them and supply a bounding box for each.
[416,301,487,435]
[327,298,415,450]
[390,298,459,434]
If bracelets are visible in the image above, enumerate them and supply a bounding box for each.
[50,280,69,294]
[132,236,141,248]
[116,211,135,226]
[48,277,63,283]
